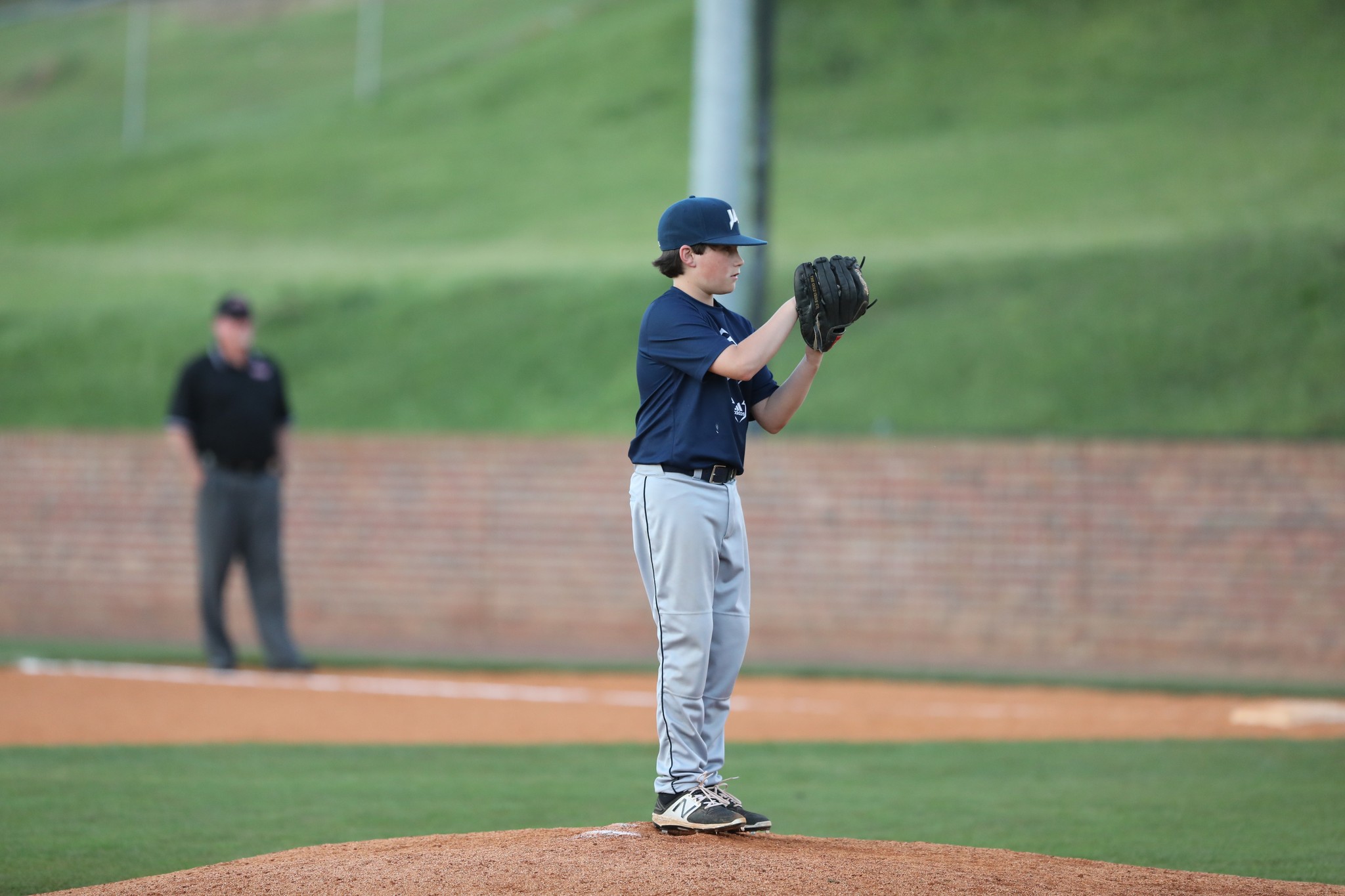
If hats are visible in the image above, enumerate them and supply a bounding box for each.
[657,196,768,251]
[218,296,249,320]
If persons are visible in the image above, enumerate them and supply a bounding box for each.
[625,197,869,835]
[167,297,315,674]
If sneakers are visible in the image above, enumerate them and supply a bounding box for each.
[704,777,772,834]
[651,770,746,834]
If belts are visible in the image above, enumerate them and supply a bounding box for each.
[661,462,737,485]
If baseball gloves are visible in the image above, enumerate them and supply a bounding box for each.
[793,254,869,353]
